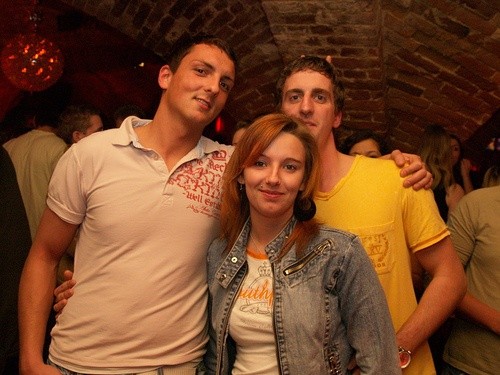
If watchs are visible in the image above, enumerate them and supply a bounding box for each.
[398,346,412,369]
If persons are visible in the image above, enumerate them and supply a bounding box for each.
[0,107,146,375]
[18,31,434,375]
[272,55,468,375]
[343,125,499,375]
[51,114,401,375]
[231,124,250,145]
